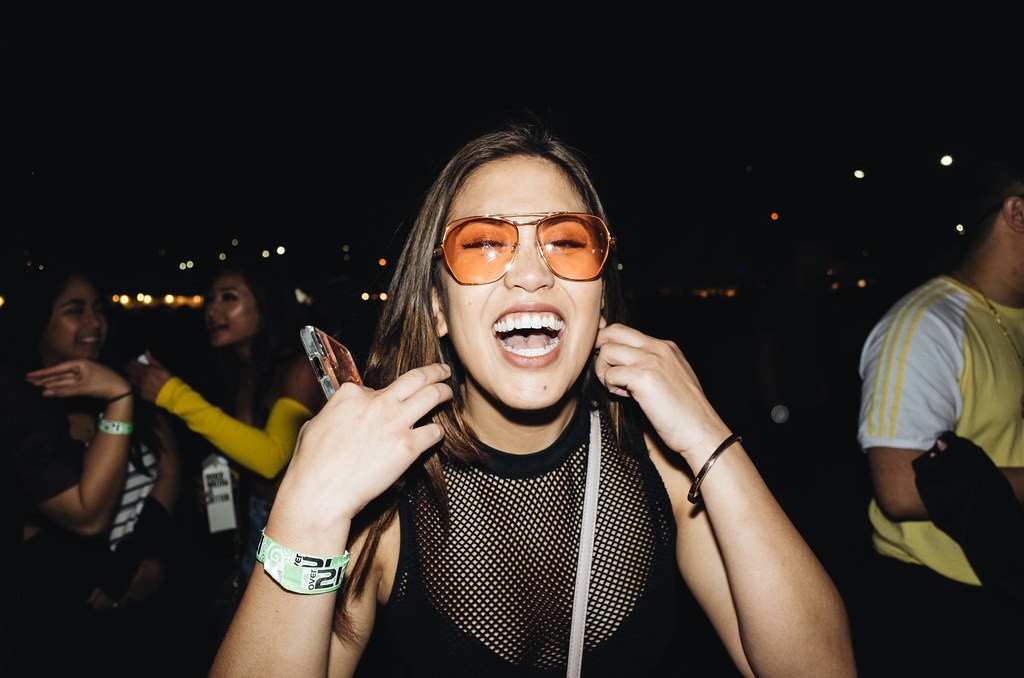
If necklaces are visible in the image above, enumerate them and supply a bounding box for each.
[957,266,1024,364]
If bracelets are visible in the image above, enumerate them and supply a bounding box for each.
[95,412,134,436]
[255,524,352,597]
[107,389,135,404]
[686,427,743,508]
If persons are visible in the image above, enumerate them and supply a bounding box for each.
[208,132,856,678]
[857,179,1024,591]
[126,257,324,606]
[0,259,172,599]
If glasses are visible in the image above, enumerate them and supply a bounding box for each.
[431,211,616,286]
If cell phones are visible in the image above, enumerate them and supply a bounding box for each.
[299,325,364,402]
